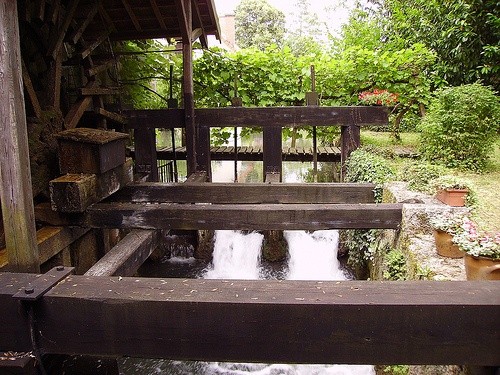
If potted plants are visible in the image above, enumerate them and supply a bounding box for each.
[430,211,473,258]
[429,175,479,207]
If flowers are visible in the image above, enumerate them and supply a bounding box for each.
[452,217,500,260]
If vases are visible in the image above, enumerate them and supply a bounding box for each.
[463,250,500,281]
